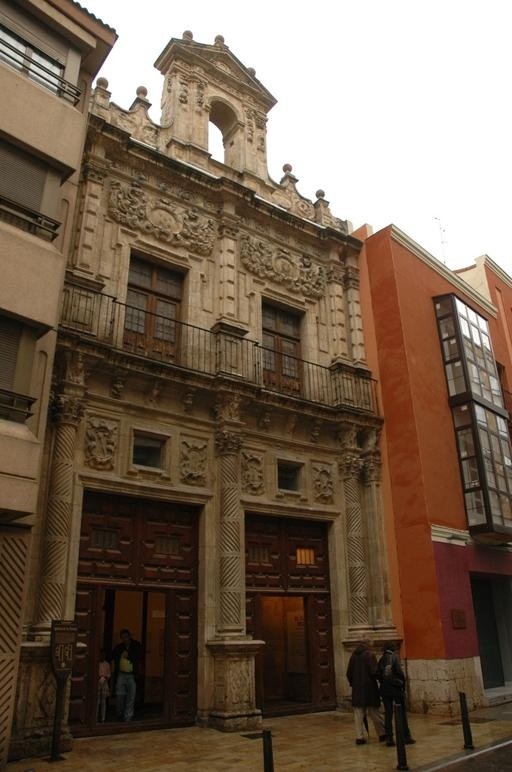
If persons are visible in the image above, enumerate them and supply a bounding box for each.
[346,645,416,747]
[97,629,141,723]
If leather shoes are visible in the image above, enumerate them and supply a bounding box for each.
[405,737,416,744]
[356,739,366,745]
[379,735,394,746]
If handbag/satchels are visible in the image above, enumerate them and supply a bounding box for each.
[383,655,406,687]
